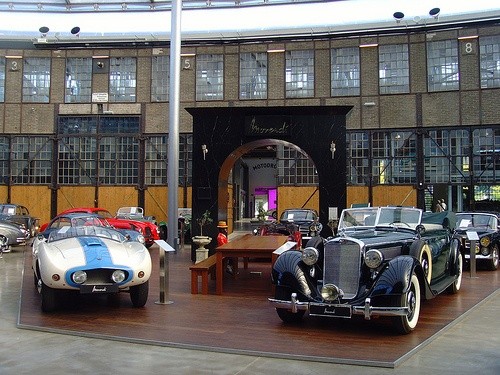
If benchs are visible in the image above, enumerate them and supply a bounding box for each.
[189,252,230,295]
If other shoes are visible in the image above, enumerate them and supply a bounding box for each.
[226,269,234,276]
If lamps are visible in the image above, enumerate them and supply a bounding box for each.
[180,52,196,56]
[458,33,479,39]
[202,144,208,160]
[330,141,336,159]
[429,8,440,22]
[92,56,110,58]
[267,48,285,53]
[359,42,378,47]
[71,27,79,39]
[5,56,22,58]
[393,12,404,25]
[40,26,49,38]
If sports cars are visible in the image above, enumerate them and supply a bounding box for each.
[252,217,304,252]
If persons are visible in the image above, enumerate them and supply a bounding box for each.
[436,200,443,213]
[287,222,302,251]
[441,199,447,211]
[217,221,232,272]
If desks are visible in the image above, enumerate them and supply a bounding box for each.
[216,235,289,295]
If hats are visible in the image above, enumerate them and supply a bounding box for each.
[217,221,228,227]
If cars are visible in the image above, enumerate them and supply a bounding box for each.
[279,208,323,239]
[0,203,191,315]
[453,210,500,271]
[268,204,463,336]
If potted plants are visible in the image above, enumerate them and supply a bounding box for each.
[192,210,213,249]
